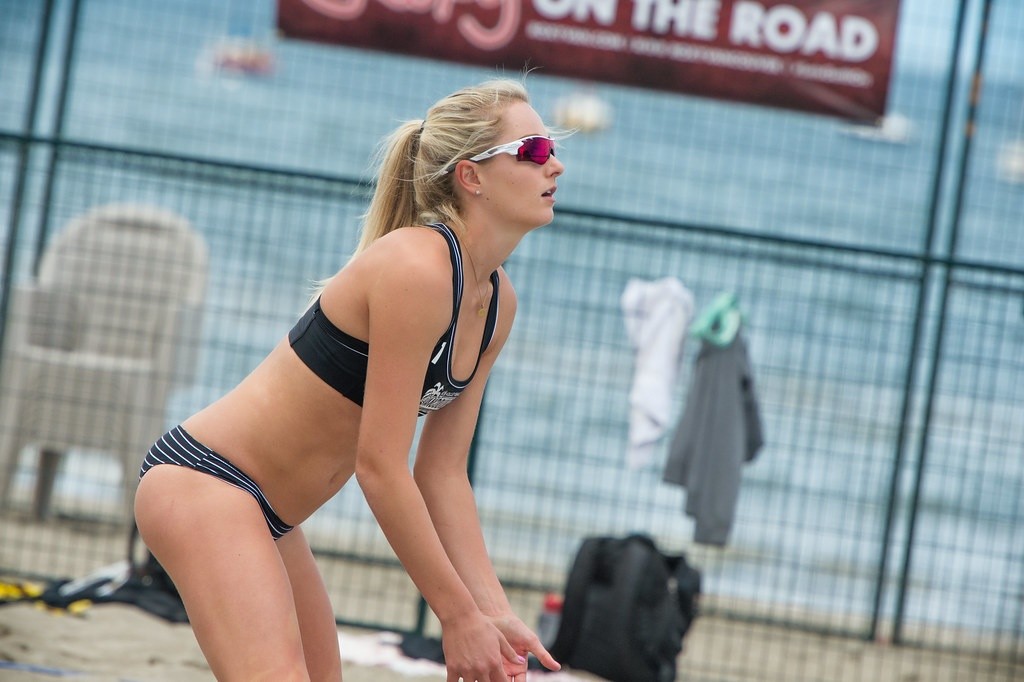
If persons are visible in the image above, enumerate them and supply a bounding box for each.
[132,78,565,682]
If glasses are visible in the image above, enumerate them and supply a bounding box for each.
[442,136,556,175]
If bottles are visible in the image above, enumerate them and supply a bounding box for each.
[537,594,564,652]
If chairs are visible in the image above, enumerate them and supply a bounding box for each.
[0,202,207,521]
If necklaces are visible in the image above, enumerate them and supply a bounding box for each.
[463,242,489,316]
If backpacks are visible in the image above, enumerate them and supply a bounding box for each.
[541,533,699,682]
